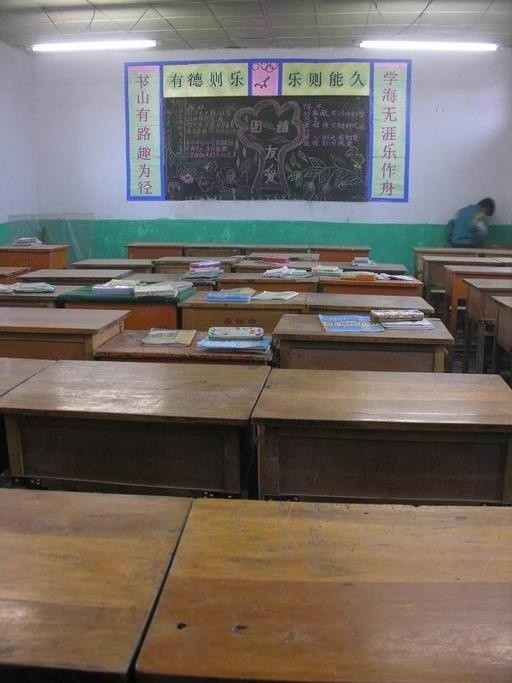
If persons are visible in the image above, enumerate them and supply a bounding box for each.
[443,197,496,249]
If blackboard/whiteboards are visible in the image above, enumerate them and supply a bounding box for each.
[163,96,368,202]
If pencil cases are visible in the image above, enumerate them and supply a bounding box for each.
[371,309,425,322]
[207,292,251,302]
[208,326,264,341]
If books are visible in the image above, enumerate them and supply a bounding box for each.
[196,334,272,354]
[13,237,42,247]
[262,264,344,281]
[91,276,195,299]
[0,281,56,295]
[375,272,416,281]
[249,290,299,302]
[318,313,385,332]
[142,327,197,346]
[370,309,435,331]
[352,256,378,268]
[206,286,255,302]
[183,261,226,279]
